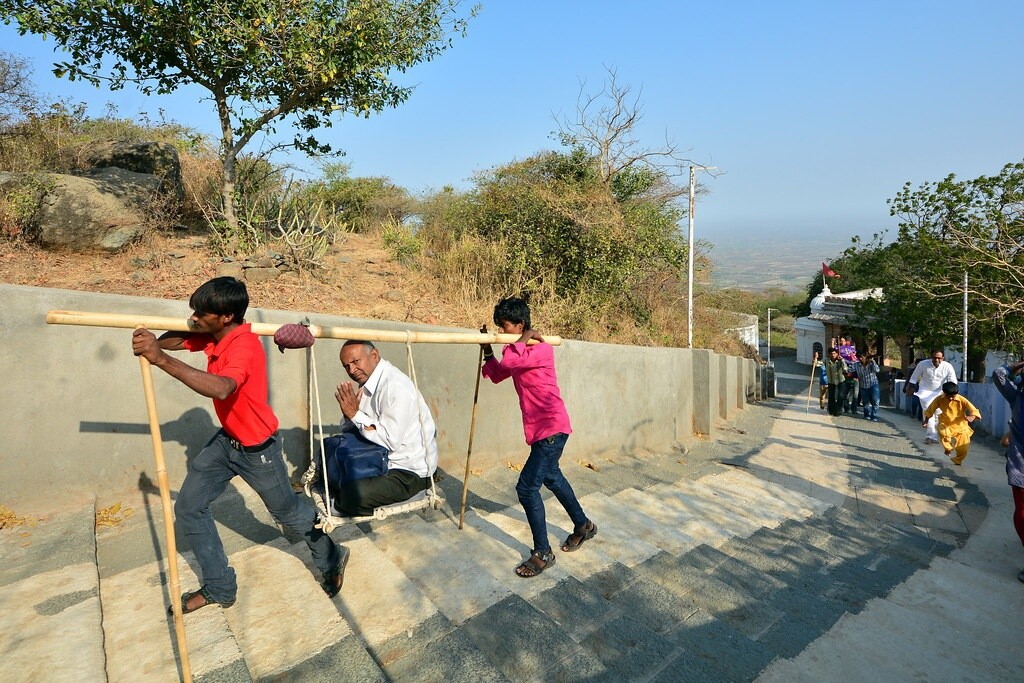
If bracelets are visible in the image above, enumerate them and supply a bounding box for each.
[484,351,493,356]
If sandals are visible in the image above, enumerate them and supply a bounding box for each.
[321,545,350,597]
[563,518,598,552]
[169,584,236,614]
[516,547,557,578]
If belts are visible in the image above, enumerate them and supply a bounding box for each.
[223,428,280,453]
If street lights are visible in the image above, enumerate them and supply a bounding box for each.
[767,308,778,368]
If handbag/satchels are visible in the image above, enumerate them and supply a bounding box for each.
[317,426,390,491]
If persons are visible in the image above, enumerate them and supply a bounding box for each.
[478,297,598,578]
[885,368,905,405]
[903,358,926,422]
[132,275,351,614]
[906,344,958,444]
[992,360,1024,584]
[812,336,881,421]
[320,340,439,518]
[922,381,982,466]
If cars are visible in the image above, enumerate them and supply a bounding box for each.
[760,338,768,346]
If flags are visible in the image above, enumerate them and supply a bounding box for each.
[823,263,841,279]
